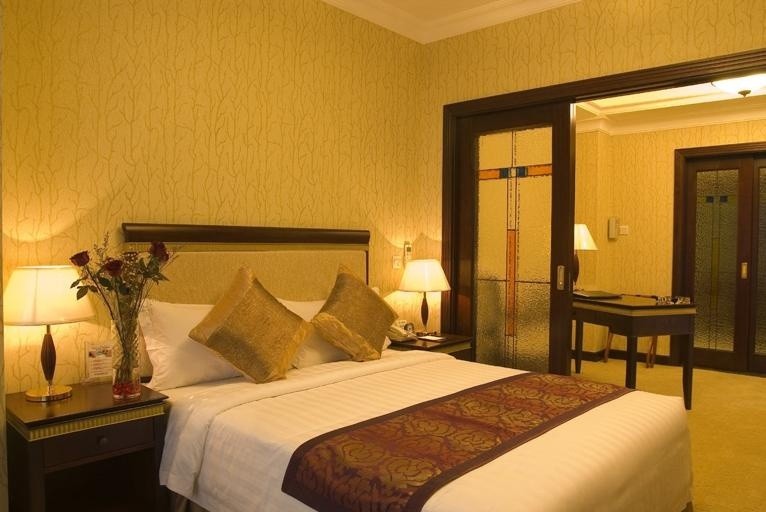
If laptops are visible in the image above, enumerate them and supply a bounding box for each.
[573,291,621,298]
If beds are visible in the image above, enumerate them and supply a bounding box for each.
[117,213,699,510]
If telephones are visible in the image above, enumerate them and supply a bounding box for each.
[386,326,417,342]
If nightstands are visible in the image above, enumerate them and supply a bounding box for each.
[389,329,473,361]
[5,372,175,512]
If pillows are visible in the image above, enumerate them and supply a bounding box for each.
[189,261,312,385]
[123,295,249,391]
[272,286,393,372]
[309,260,401,364]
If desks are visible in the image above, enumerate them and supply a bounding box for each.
[571,286,697,412]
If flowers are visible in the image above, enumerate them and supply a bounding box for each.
[71,235,174,388]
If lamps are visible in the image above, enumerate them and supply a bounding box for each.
[711,70,764,104]
[3,257,98,403]
[573,221,601,291]
[398,258,453,336]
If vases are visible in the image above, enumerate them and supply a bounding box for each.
[110,317,144,401]
